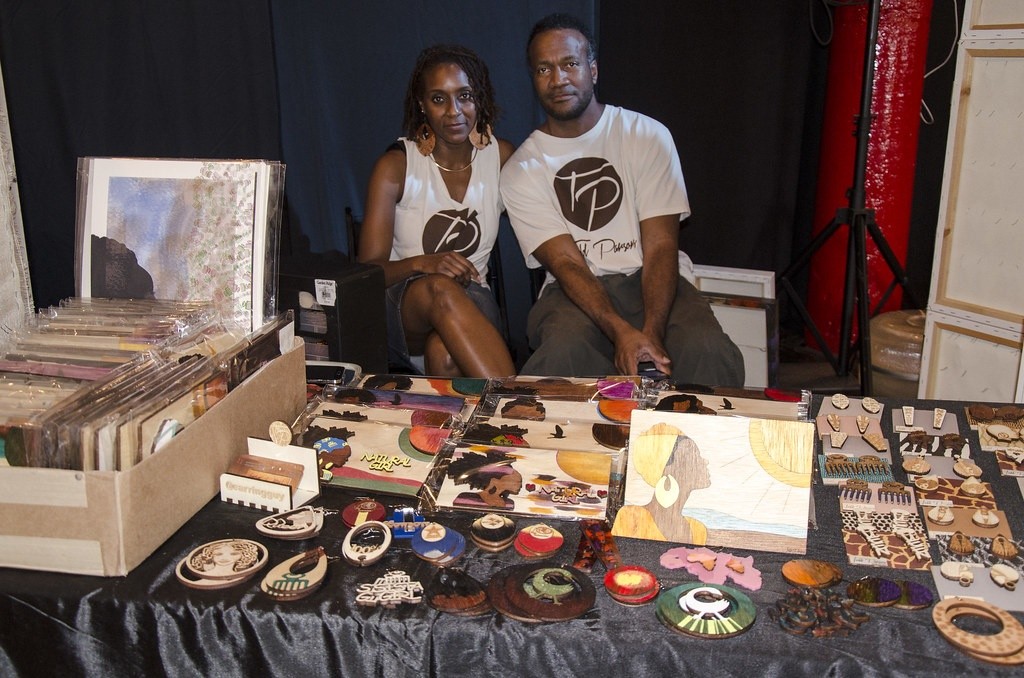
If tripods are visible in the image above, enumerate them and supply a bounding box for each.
[775,1,925,395]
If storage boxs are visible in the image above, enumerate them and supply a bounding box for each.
[0,335,306,575]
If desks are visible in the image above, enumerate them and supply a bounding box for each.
[0,375,1024,678]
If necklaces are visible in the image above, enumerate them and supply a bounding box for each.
[422,135,478,172]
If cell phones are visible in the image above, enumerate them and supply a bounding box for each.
[638,361,668,381]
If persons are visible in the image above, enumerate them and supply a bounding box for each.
[357,42,514,377]
[499,13,745,390]
